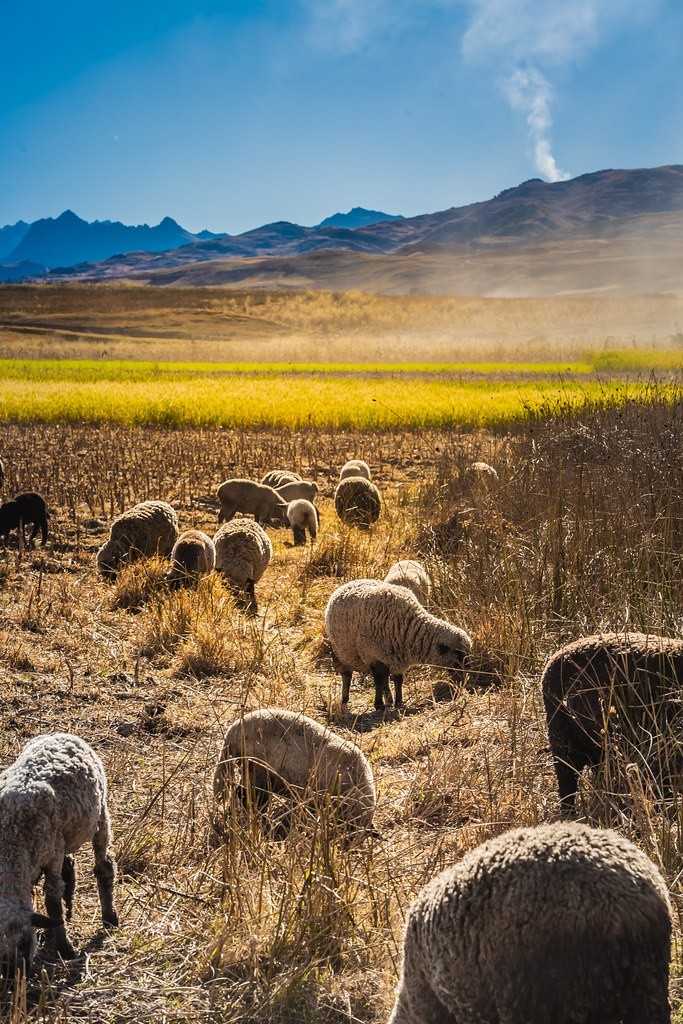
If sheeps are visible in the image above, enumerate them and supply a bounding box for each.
[340,460,370,480]
[216,479,287,523]
[277,499,318,545]
[541,632,683,820]
[261,471,302,488]
[390,820,676,1024]
[466,461,498,492]
[384,559,429,608]
[95,500,179,584]
[335,478,382,530]
[164,530,215,592]
[0,493,51,548]
[325,579,473,712]
[0,460,4,488]
[214,519,271,591]
[275,481,319,504]
[0,732,120,992]
[213,708,382,847]
